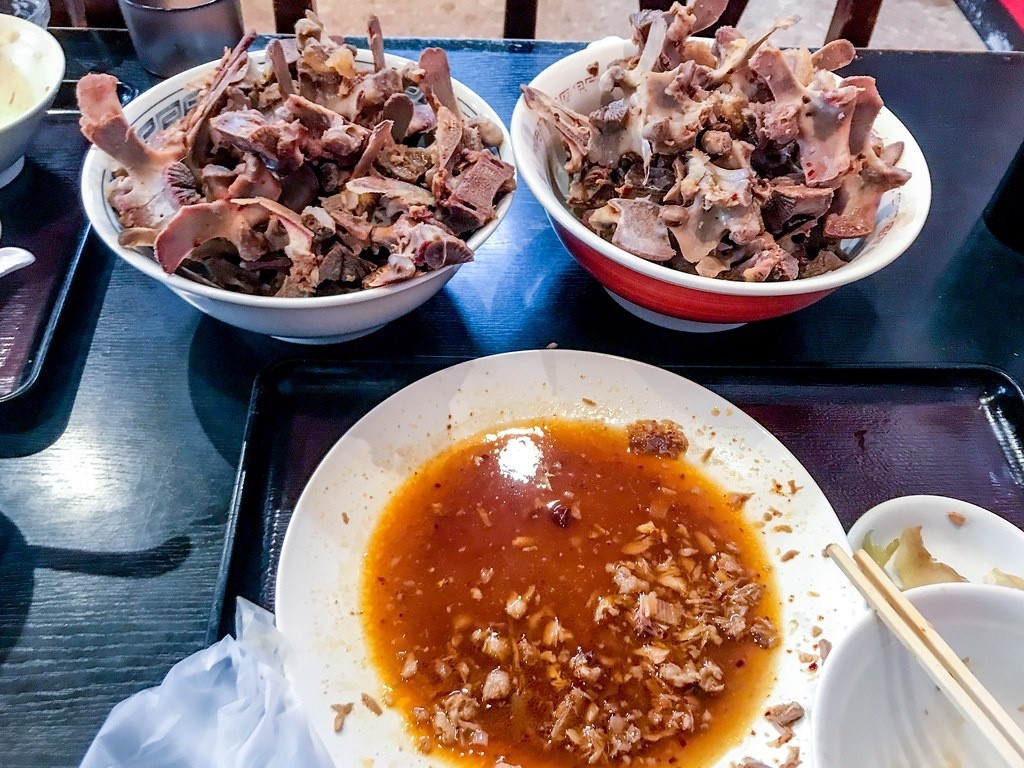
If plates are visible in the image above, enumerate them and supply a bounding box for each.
[275,349,871,768]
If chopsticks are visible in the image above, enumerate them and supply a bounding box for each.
[825,544,1024,767]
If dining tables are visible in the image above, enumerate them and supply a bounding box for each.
[0,28,1024,768]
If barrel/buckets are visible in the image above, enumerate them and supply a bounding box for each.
[118,1,243,77]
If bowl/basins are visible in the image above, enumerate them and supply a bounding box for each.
[506,33,931,332]
[80,42,517,346]
[813,581,1023,768]
[0,13,67,191]
[842,495,1024,612]
